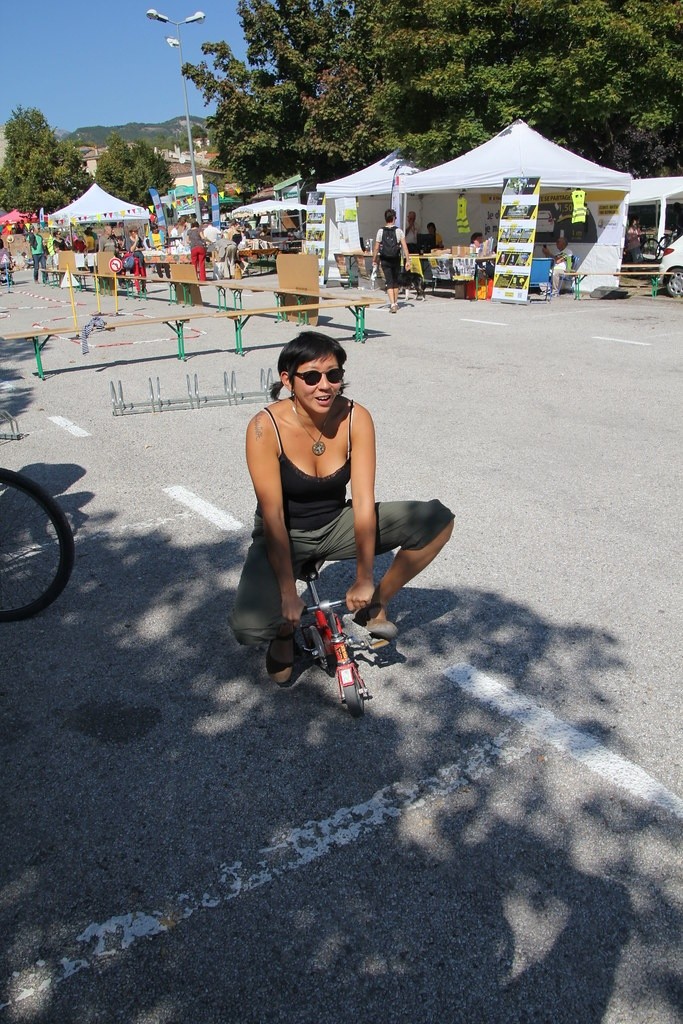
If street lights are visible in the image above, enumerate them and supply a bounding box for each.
[146,8,207,226]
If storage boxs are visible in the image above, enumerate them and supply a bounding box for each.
[529,257,554,288]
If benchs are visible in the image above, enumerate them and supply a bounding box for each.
[0,269,388,382]
[620,263,661,271]
[562,272,675,301]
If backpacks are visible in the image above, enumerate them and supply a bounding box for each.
[381,226,401,262]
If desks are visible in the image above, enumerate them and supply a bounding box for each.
[143,253,192,263]
[333,251,386,289]
[402,252,497,303]
[238,248,278,276]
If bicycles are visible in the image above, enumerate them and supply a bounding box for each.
[0,468,77,622]
[296,560,390,718]
[638,229,678,262]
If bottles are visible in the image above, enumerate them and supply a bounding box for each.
[365,242,370,251]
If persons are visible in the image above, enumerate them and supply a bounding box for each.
[627,214,644,279]
[673,201,683,237]
[0,214,272,292]
[542,238,574,298]
[229,331,456,685]
[372,209,496,314]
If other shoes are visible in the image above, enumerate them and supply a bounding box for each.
[355,603,398,638]
[266,632,293,682]
[545,289,558,293]
[391,303,398,313]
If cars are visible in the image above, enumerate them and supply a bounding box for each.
[659,235,683,297]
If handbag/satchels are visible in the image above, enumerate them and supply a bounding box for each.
[43,245,49,255]
[122,251,134,268]
[235,263,242,279]
[53,253,59,265]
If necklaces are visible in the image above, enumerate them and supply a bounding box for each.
[289,396,329,456]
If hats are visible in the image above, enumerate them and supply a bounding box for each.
[34,225,40,228]
[129,225,138,231]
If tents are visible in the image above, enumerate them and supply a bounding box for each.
[233,200,307,240]
[0,208,48,225]
[316,116,683,292]
[49,182,151,271]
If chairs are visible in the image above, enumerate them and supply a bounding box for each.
[552,256,580,296]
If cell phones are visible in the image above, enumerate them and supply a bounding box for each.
[543,244,546,248]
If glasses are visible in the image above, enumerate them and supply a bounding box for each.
[295,369,345,386]
[58,233,61,235]
